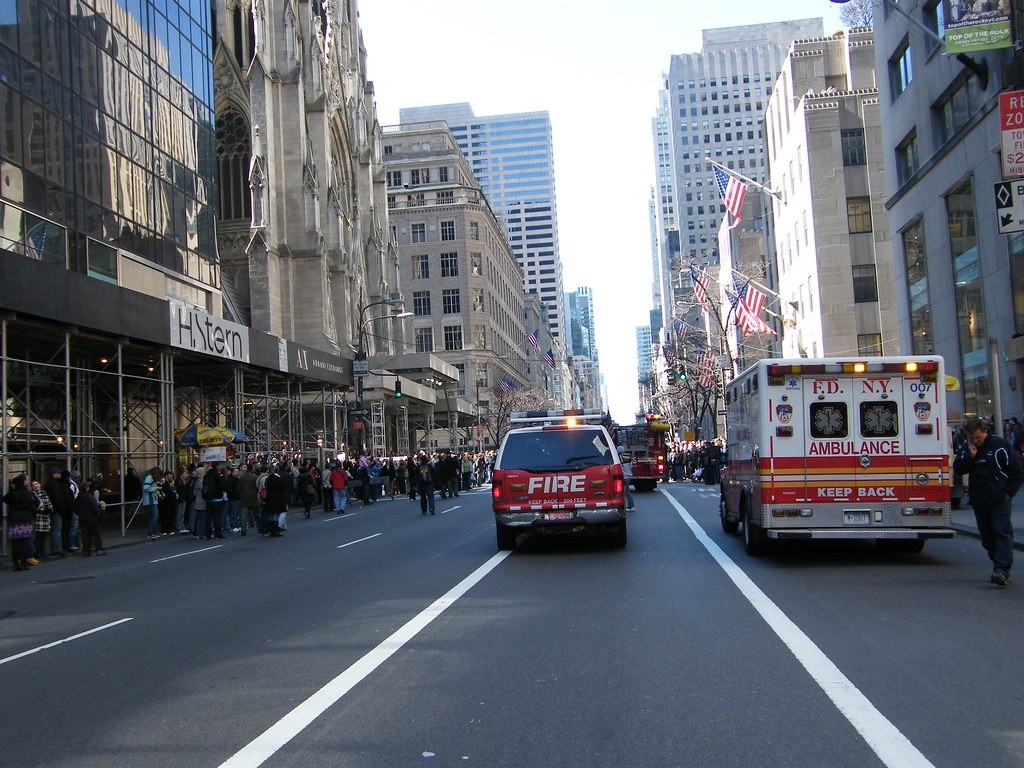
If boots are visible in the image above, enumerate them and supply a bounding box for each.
[12,559,30,572]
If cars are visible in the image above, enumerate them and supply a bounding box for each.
[491,408,626,550]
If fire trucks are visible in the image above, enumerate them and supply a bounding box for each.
[614,414,670,491]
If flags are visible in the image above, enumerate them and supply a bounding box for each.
[543,348,557,370]
[691,266,711,318]
[714,165,749,230]
[662,315,718,388]
[528,329,539,355]
[725,272,778,338]
[497,374,521,396]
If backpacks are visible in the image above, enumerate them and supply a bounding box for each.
[418,466,432,483]
[201,472,216,500]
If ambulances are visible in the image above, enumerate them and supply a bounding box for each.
[718,355,959,557]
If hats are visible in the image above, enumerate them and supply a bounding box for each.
[80,481,92,486]
[50,467,63,473]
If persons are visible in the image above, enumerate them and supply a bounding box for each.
[953,419,1024,585]
[951,415,1024,505]
[660,437,729,486]
[110,448,500,541]
[616,446,635,511]
[6,470,107,572]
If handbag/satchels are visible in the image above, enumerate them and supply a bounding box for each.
[259,489,266,501]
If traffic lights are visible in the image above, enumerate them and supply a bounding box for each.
[354,421,364,430]
[668,375,677,385]
[395,381,402,398]
[678,366,686,380]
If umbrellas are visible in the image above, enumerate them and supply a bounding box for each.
[178,423,250,449]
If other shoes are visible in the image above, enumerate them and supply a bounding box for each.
[70,546,79,550]
[626,507,635,511]
[153,534,161,537]
[146,535,158,539]
[163,526,250,539]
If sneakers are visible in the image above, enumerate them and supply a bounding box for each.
[26,557,39,565]
[991,573,1013,583]
[82,551,91,557]
[96,550,105,556]
[993,573,1007,585]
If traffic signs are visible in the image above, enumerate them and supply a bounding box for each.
[351,409,369,416]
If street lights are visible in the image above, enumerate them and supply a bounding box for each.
[357,298,416,456]
